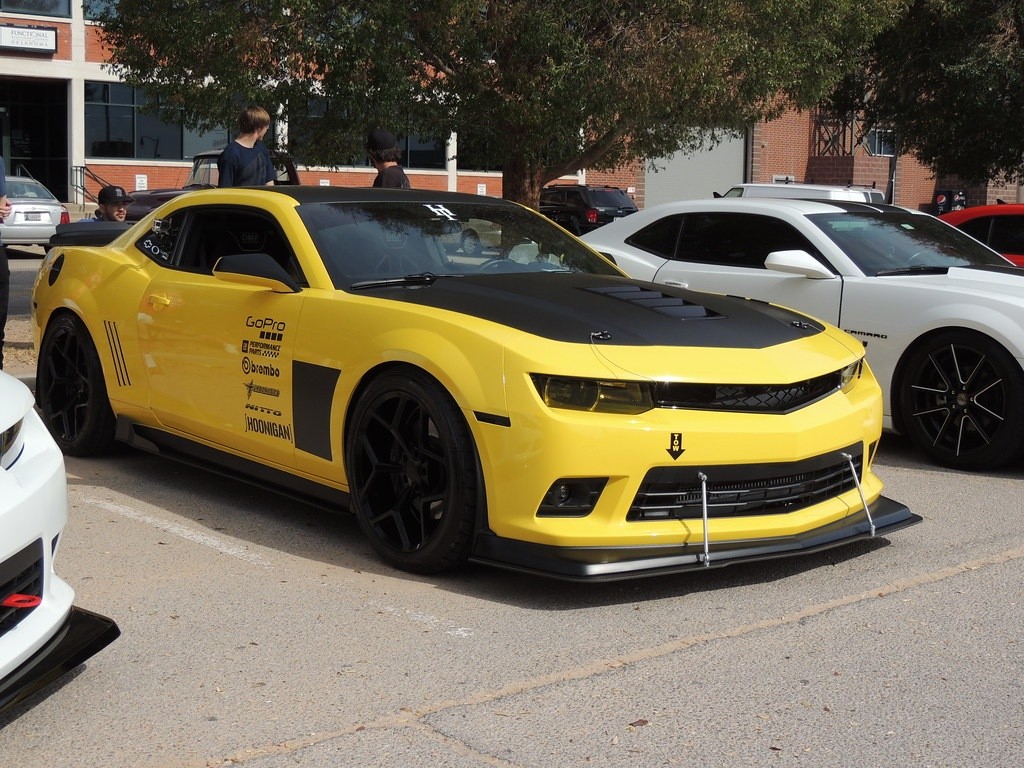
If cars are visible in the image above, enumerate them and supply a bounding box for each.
[861,190,885,203]
[0,368,122,713]
[573,198,1024,474]
[31,182,925,585]
[0,176,70,255]
[126,150,300,226]
[936,199,1024,269]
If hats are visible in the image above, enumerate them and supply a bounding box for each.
[368,125,395,151]
[98,185,135,205]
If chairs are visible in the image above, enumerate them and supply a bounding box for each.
[200,230,302,288]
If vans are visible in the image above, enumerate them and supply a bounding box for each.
[713,183,867,203]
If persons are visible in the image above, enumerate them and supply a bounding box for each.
[366,129,410,189]
[217,105,277,188]
[0,156,10,370]
[79,186,135,223]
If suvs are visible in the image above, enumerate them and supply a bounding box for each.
[539,183,639,237]
